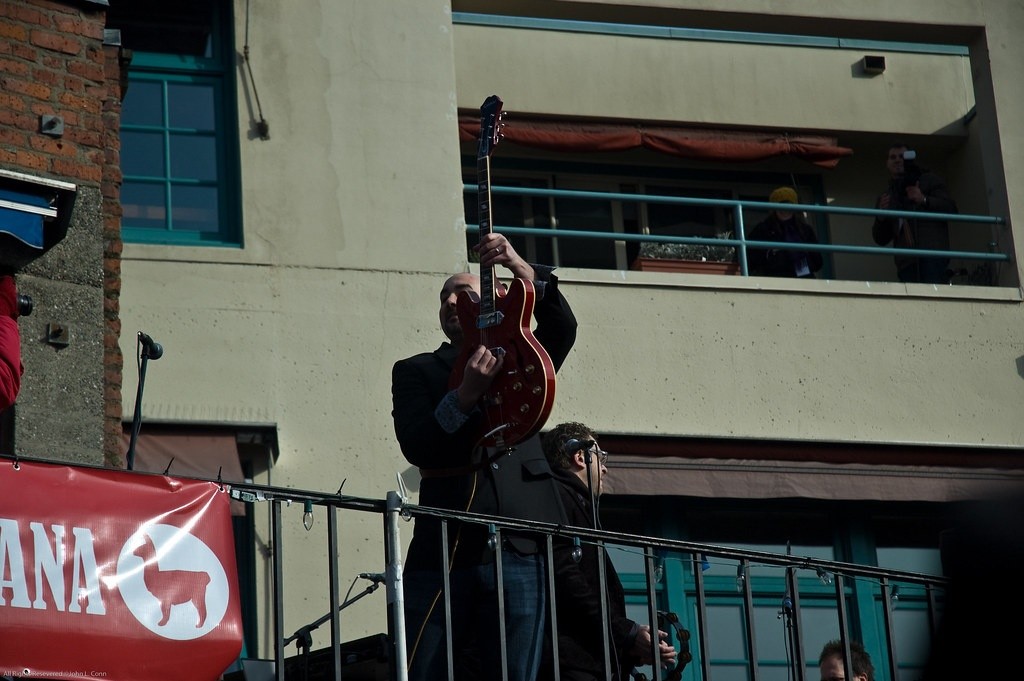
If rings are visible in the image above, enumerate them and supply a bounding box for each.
[495,248,500,255]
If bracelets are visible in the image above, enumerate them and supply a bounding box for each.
[923,195,928,206]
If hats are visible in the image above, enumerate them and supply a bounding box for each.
[770,187,798,203]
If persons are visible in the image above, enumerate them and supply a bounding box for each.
[734,186,823,280]
[0,273,25,407]
[543,420,679,681]
[391,233,577,681]
[872,141,958,284]
[819,639,876,681]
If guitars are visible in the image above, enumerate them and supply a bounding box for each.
[447,92,556,453]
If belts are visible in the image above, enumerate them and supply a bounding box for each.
[486,536,514,551]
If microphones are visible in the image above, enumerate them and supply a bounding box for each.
[565,438,595,453]
[358,572,386,585]
[137,331,163,359]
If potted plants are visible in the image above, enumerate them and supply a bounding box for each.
[633,226,740,275]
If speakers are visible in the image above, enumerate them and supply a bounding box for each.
[222,633,390,681]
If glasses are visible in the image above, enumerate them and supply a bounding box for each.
[588,448,608,464]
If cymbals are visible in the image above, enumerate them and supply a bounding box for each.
[629,609,692,681]
